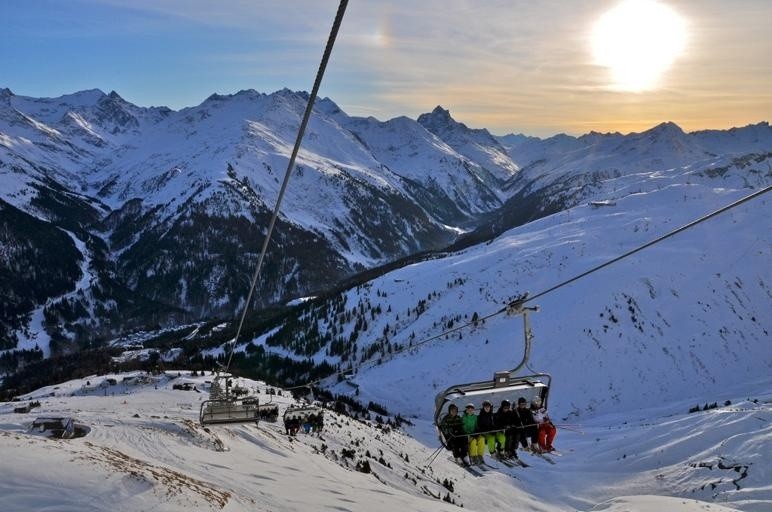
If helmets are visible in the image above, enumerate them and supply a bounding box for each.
[449,396,542,416]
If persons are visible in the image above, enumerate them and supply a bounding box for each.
[461,403,486,465]
[285,412,324,435]
[528,396,556,454]
[495,399,520,458]
[513,397,539,455]
[260,407,278,420]
[478,400,506,460]
[243,399,257,405]
[440,403,469,465]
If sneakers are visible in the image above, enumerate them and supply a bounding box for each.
[455,445,554,466]
